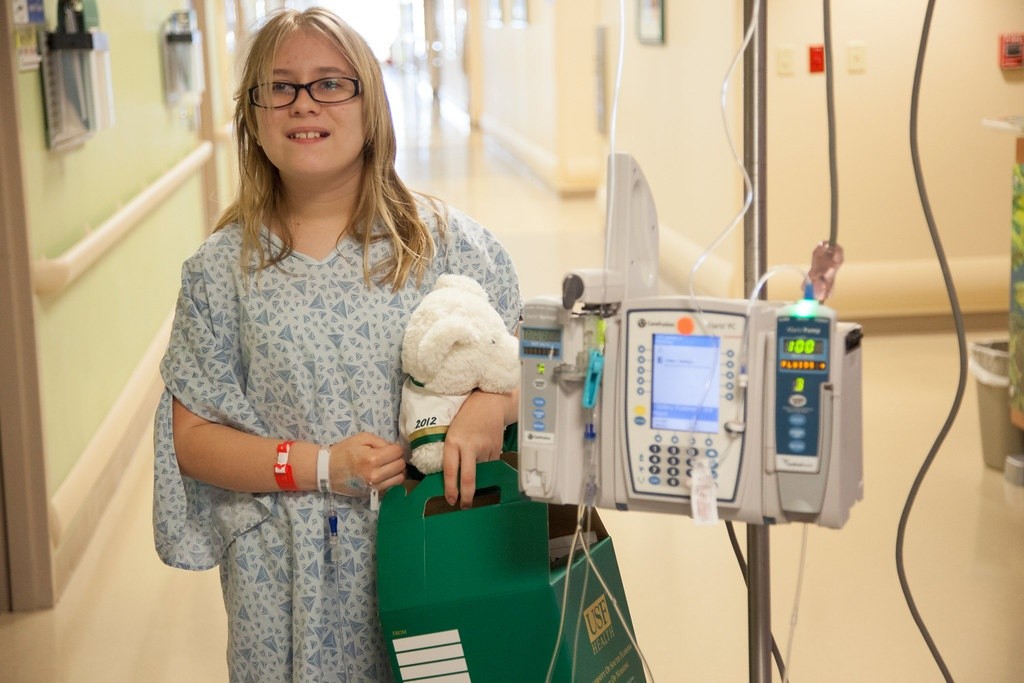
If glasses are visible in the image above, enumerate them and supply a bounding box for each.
[247,75,361,109]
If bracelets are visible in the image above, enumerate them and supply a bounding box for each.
[273,440,301,492]
[316,443,334,493]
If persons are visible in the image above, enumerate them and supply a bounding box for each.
[152,6,523,683]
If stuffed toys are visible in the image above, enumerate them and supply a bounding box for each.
[397,273,520,475]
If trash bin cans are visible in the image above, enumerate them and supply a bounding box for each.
[969,337,1024,474]
